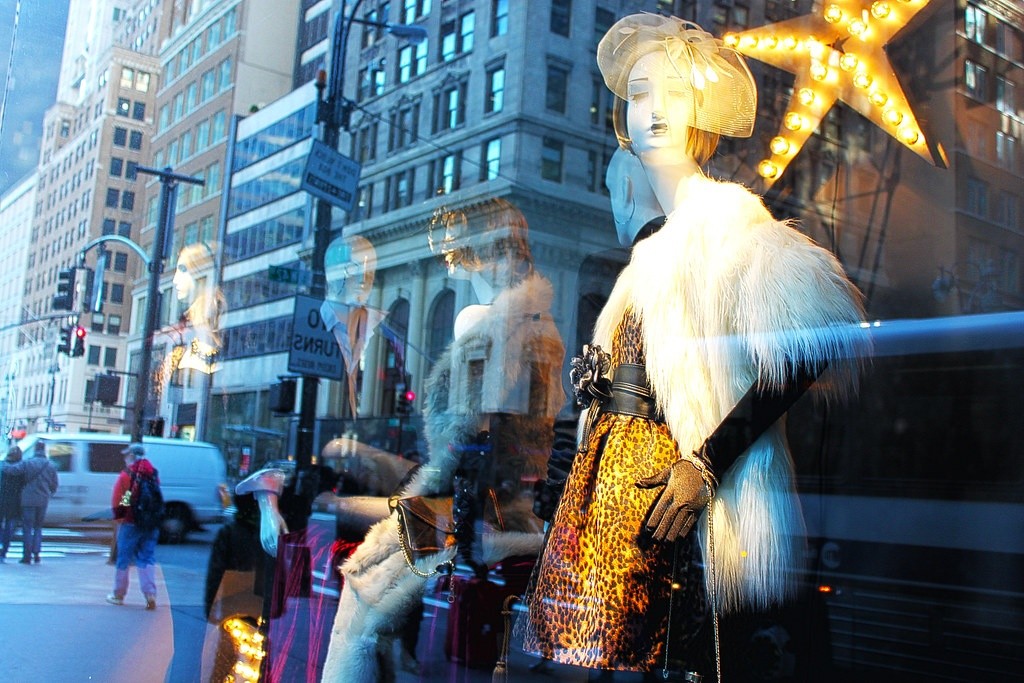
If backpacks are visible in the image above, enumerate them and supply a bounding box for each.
[122,467,165,530]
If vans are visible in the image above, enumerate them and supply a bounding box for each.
[669,310,1023,682]
[2,431,228,546]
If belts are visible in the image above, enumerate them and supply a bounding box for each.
[594,363,666,416]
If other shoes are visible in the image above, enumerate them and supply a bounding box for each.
[147,596,156,610]
[19,558,32,564]
[35,556,41,563]
[107,594,123,605]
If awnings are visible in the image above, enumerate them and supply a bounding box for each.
[227,424,287,442]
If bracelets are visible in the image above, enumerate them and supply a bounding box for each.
[684,453,719,682]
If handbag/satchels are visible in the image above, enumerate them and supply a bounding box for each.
[388,487,505,576]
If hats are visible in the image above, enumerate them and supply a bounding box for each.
[121,442,145,455]
[5,446,22,462]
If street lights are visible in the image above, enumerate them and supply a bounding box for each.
[45,363,61,433]
[285,14,429,497]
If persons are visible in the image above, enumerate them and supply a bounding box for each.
[147,238,237,415]
[503,11,873,683]
[106,442,162,610]
[236,232,426,559]
[0,440,59,564]
[309,198,565,674]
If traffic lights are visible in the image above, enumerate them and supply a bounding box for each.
[72,326,86,357]
[83,269,104,314]
[53,269,76,311]
[56,326,73,355]
[397,390,414,413]
